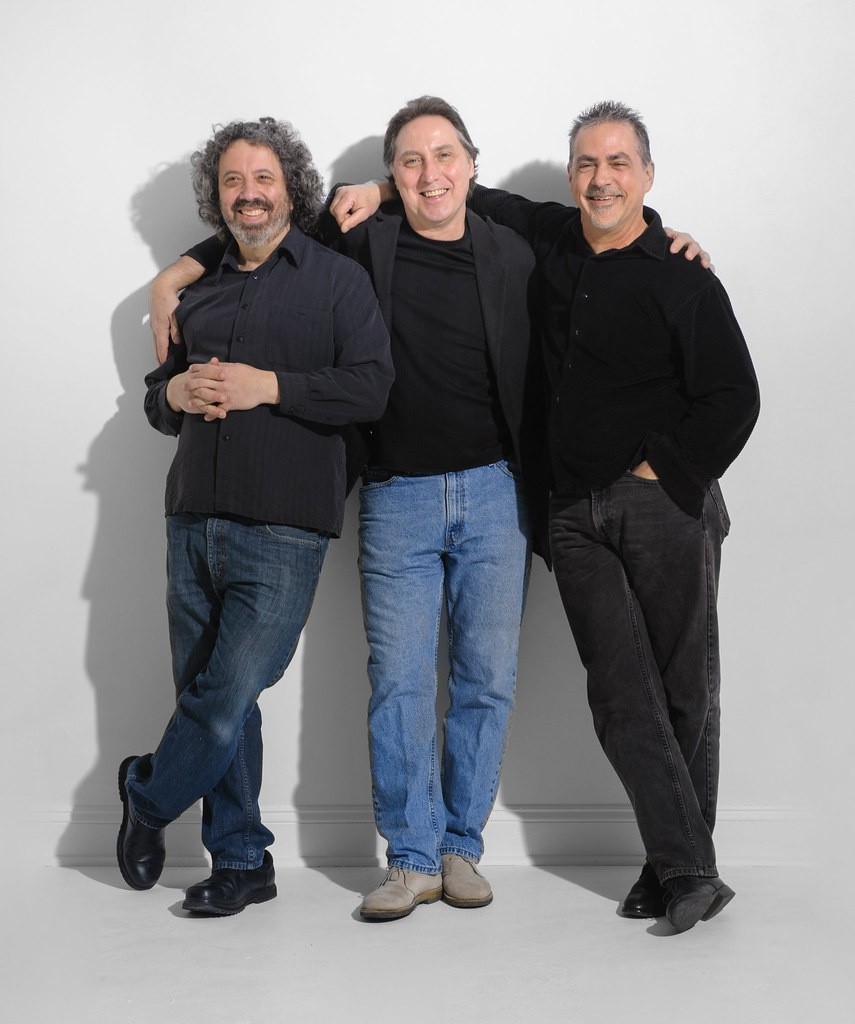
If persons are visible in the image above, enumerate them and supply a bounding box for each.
[149,93,715,923]
[116,118,397,911]
[323,102,735,934]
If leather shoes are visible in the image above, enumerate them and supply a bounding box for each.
[665,874,735,934]
[360,866,443,919]
[182,850,277,914]
[116,754,166,891]
[621,862,665,918]
[442,853,493,908]
